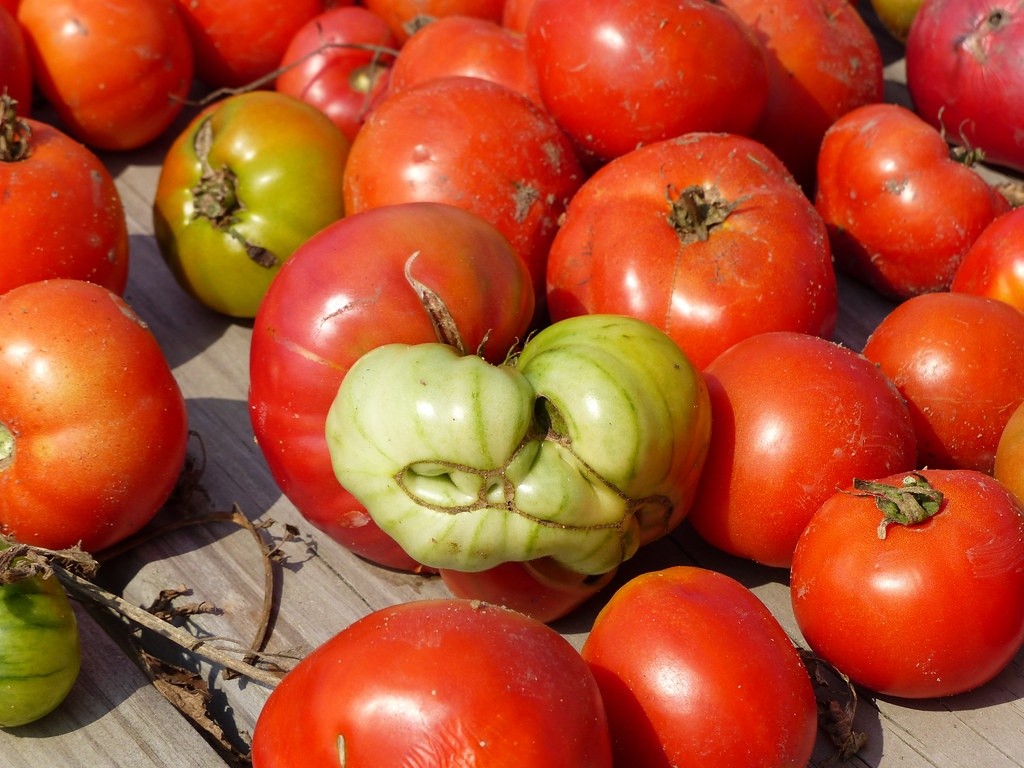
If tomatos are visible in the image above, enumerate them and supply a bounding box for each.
[0,0,1024,767]
